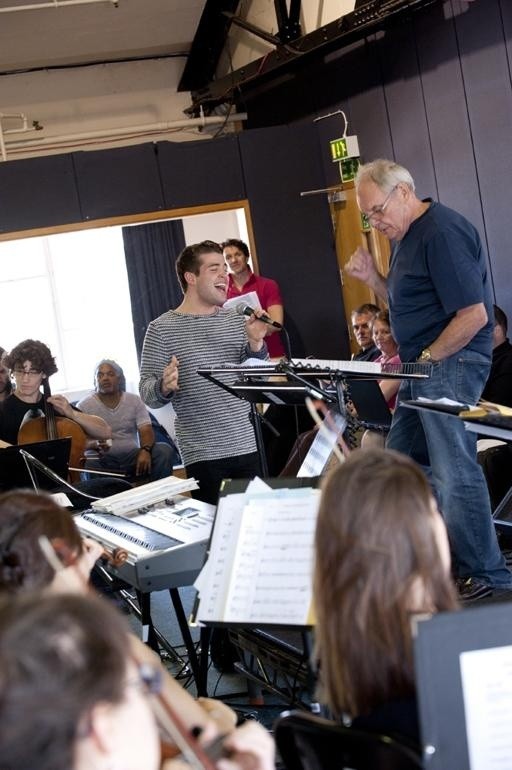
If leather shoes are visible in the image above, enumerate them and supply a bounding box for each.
[455,577,495,605]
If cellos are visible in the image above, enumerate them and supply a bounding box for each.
[19,377,87,481]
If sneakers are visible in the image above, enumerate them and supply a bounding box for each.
[212,659,246,676]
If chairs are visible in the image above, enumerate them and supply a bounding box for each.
[275,706,426,768]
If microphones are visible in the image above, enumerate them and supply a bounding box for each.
[236,302,280,330]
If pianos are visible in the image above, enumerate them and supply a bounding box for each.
[69,496,217,594]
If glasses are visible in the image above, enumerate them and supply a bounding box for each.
[10,368,42,378]
[362,186,398,226]
